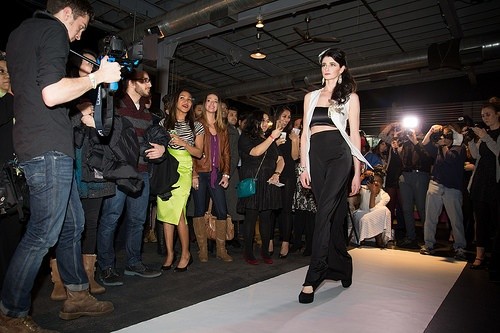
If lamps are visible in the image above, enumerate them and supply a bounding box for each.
[250,33,266,60]
[255,6,264,28]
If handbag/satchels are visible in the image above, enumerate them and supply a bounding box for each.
[205,211,235,242]
[236,179,257,198]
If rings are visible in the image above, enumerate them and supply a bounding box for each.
[197,185,199,187]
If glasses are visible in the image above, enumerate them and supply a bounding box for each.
[137,78,152,84]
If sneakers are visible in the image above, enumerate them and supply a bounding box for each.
[455,248,467,260]
[420,241,435,254]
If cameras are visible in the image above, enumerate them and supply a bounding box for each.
[366,175,374,183]
[430,131,446,144]
[98,34,125,57]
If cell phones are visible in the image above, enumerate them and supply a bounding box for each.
[275,119,281,129]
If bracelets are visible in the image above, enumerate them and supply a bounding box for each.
[274,172,280,175]
[223,175,229,178]
[298,167,305,175]
[270,135,274,141]
[89,73,96,89]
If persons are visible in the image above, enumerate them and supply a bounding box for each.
[0,53,31,290]
[299,49,374,304]
[97,69,163,287]
[155,89,315,272]
[0,0,121,333]
[468,98,500,268]
[360,116,482,260]
[51,48,106,300]
[352,171,392,248]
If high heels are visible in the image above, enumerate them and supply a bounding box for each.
[174,254,193,272]
[341,280,351,287]
[279,245,290,258]
[289,241,302,252]
[302,247,312,256]
[298,290,315,303]
[161,255,176,270]
[265,242,275,256]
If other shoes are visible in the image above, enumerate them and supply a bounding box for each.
[471,257,486,269]
[244,255,258,265]
[125,262,161,278]
[398,239,416,247]
[260,254,272,263]
[99,267,124,286]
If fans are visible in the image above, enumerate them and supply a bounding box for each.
[287,16,341,50]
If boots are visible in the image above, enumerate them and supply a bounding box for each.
[50,258,66,301]
[144,207,159,243]
[84,254,106,294]
[214,218,232,262]
[157,223,177,255]
[59,288,114,319]
[0,312,61,333]
[193,216,209,263]
